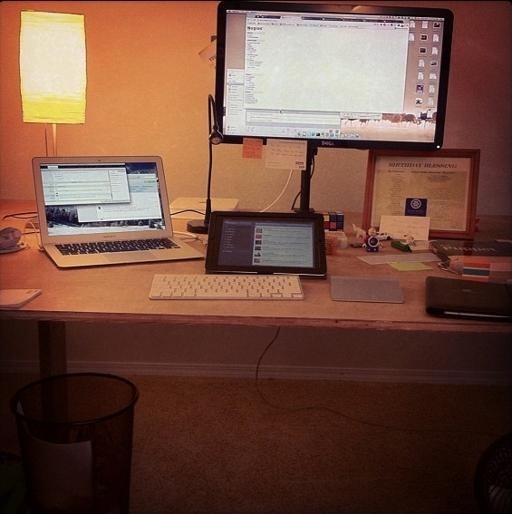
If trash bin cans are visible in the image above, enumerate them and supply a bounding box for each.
[9,372,140,514]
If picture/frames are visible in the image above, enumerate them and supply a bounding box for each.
[363,148,480,241]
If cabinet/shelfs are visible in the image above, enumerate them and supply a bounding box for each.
[0,199,511,513]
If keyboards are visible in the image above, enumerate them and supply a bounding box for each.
[148,272,305,301]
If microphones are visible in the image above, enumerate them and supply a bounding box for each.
[186,93,224,232]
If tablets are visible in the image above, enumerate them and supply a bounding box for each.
[205,210,328,277]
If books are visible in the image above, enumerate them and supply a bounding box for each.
[425,274,511,327]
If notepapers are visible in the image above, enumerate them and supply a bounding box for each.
[462,258,490,282]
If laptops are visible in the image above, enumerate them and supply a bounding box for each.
[30,156,204,269]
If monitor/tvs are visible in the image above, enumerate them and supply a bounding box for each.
[213,0,456,153]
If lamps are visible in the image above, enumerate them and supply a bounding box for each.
[20,10,88,229]
